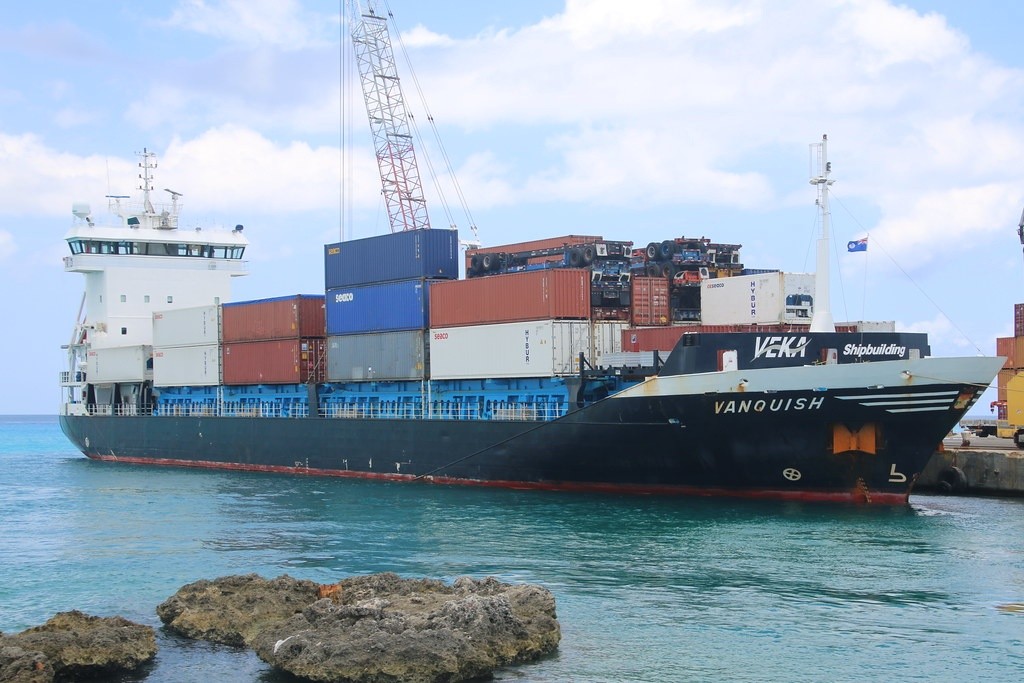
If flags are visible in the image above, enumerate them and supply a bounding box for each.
[847,236,868,252]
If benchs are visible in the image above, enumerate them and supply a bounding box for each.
[495,406,534,420]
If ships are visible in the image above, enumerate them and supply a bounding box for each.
[58,0,1009,510]
